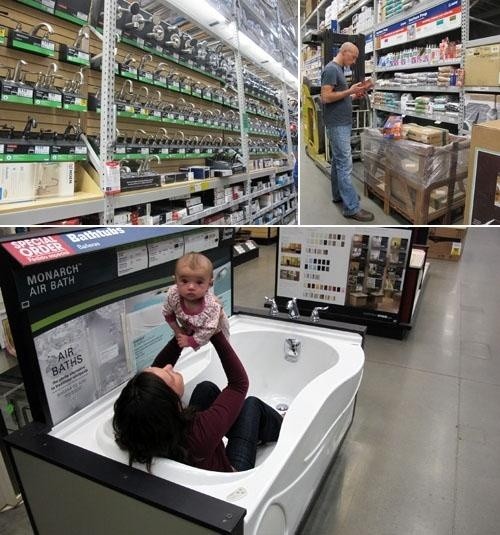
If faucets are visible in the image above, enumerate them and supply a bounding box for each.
[263,296,279,316]
[287,298,300,320]
[310,306,328,324]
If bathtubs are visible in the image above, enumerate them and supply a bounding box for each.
[49,313,365,535]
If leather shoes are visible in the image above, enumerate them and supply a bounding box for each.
[334,193,374,221]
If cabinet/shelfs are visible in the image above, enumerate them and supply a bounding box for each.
[302,0,500,135]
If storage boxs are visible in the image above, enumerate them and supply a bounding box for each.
[408,126,449,146]
[463,119,500,225]
[426,228,467,261]
[465,44,500,87]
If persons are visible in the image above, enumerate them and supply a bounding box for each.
[161,251,230,350]
[111,322,283,472]
[321,44,375,221]
